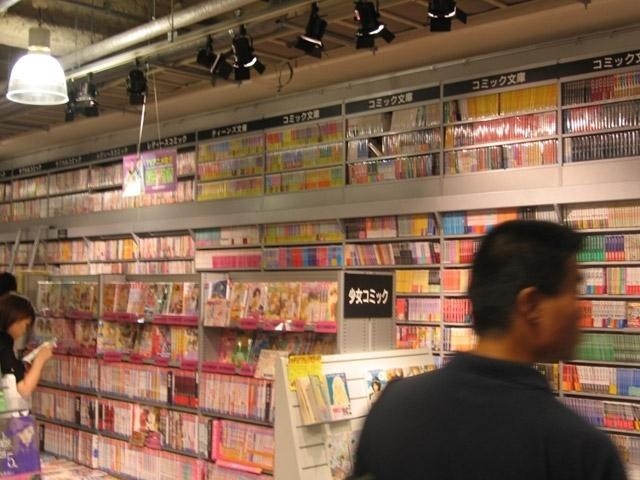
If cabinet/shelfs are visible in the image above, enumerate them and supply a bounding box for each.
[191,224,263,273]
[1,236,134,275]
[339,211,443,354]
[19,271,126,467]
[437,204,561,398]
[273,351,437,480]
[0,50,640,224]
[260,216,342,269]
[134,234,195,275]
[97,274,223,480]
[199,271,394,480]
[557,199,640,480]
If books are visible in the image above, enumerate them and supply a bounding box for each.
[1,236,196,296]
[206,280,353,480]
[345,200,639,328]
[196,219,344,268]
[22,281,208,480]
[0,146,196,222]
[607,433,639,480]
[531,332,639,433]
[196,118,344,202]
[397,325,478,369]
[347,72,639,184]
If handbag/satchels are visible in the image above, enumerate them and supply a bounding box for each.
[0,407,42,479]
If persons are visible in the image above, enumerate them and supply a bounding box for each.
[1,292,55,418]
[344,220,630,480]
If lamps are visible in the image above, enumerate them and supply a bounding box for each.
[5,1,72,107]
[196,0,469,82]
